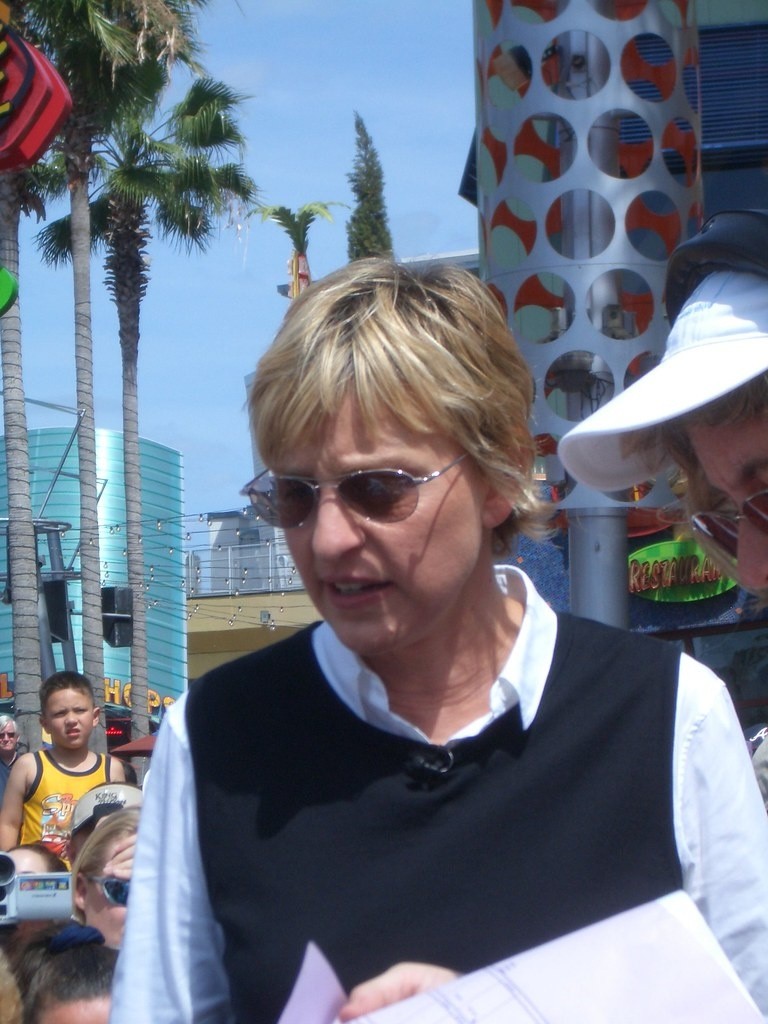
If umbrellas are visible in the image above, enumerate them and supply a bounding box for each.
[110,734,156,778]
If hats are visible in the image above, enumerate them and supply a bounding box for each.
[557,269,768,493]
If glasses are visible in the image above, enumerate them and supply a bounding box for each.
[87,875,129,904]
[691,489,768,558]
[238,452,470,528]
[0,733,17,738]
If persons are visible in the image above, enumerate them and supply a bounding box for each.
[0,781,142,1024]
[110,256,768,1024]
[0,671,125,872]
[0,715,25,807]
[557,208,768,616]
